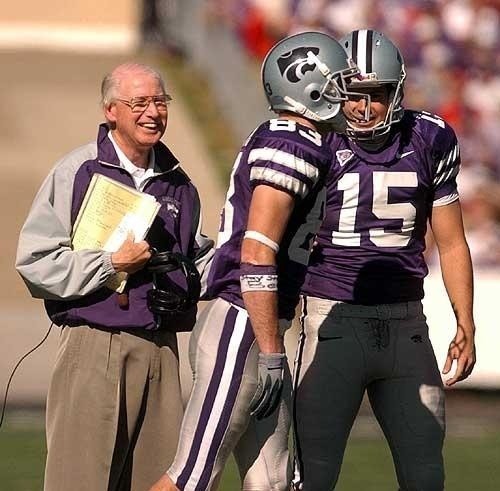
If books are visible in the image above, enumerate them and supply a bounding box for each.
[70,170,162,294]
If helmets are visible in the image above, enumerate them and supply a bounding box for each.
[339,29,404,141]
[261,30,354,122]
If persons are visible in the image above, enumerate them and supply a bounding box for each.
[12,61,214,491]
[216,2,500,279]
[293,27,479,490]
[145,33,355,491]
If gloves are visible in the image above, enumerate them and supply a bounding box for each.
[247,352,287,419]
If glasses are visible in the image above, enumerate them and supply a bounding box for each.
[109,95,171,112]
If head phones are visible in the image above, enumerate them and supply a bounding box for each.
[145,251,200,318]
